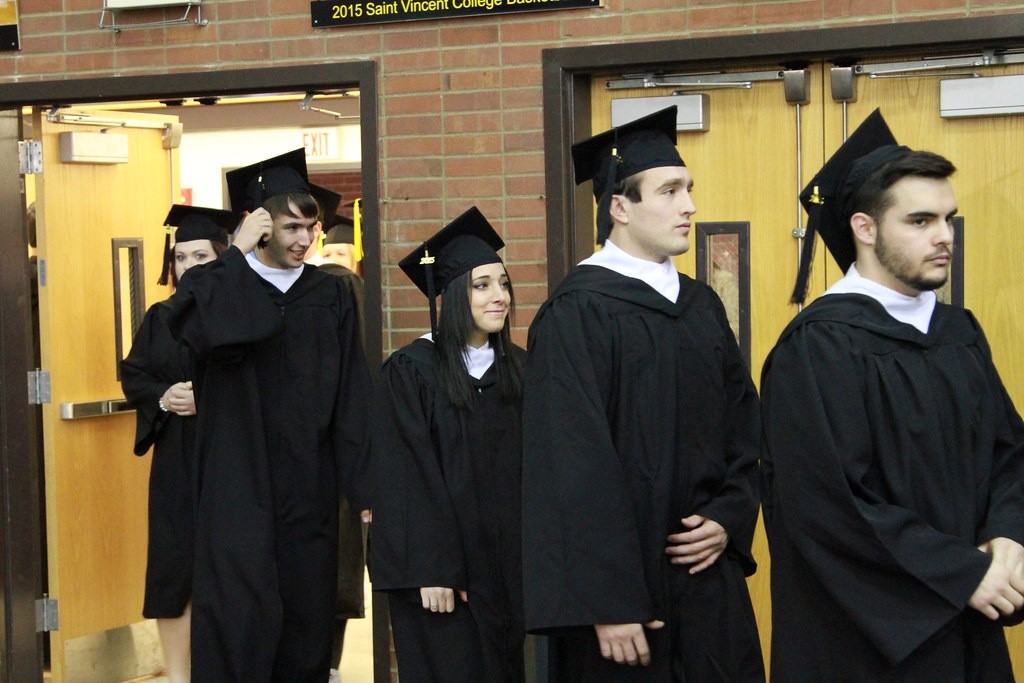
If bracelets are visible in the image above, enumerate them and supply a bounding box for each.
[158,398,168,412]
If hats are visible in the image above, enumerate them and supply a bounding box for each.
[570,105,686,245]
[397,207,504,343]
[226,149,310,250]
[325,211,356,246]
[342,198,366,263]
[789,105,911,306]
[157,204,235,286]
[308,185,341,250]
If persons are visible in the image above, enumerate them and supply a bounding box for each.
[303,180,371,682]
[119,204,244,683]
[366,206,530,683]
[169,147,372,683]
[517,105,766,682]
[758,109,1024,683]
